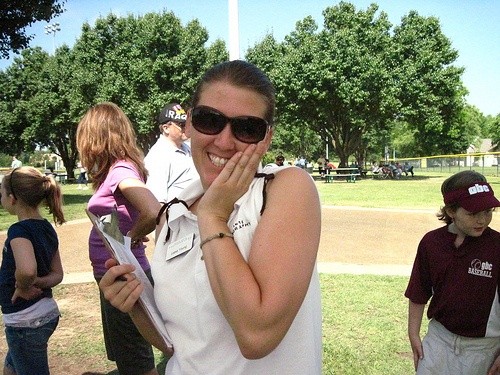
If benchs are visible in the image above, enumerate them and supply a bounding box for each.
[371,170,411,179]
[325,174,361,183]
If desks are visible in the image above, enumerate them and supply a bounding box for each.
[56,174,68,184]
[327,168,359,175]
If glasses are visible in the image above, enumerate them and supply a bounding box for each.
[190,105,271,145]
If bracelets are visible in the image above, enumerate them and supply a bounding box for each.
[200,232,235,250]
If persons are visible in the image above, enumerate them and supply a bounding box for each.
[141,102,201,244]
[77,160,89,190]
[404,170,500,375]
[11,156,23,169]
[97,59,323,375]
[75,102,163,375]
[0,166,66,375]
[276,152,414,180]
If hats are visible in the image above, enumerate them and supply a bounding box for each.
[441,181,500,213]
[158,103,189,124]
